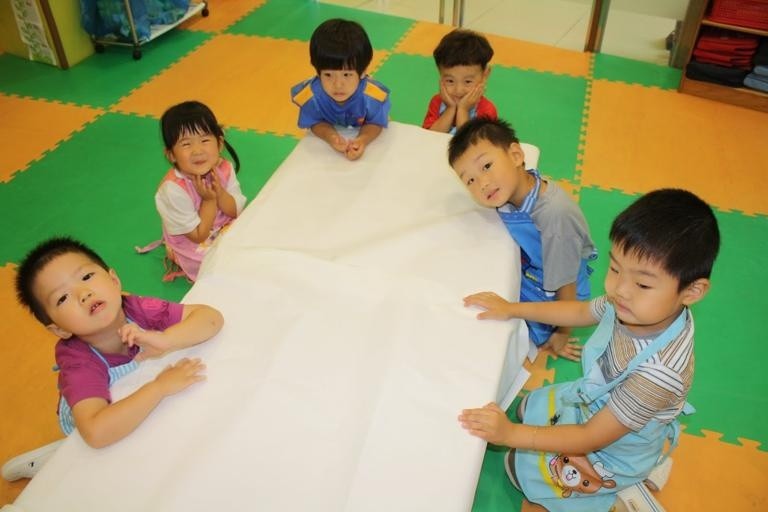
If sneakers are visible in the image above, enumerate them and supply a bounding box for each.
[1,436,70,483]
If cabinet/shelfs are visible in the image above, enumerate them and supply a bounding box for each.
[670,0,768,114]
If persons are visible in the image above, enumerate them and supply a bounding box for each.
[289,17,391,162]
[456,189,720,511]
[421,29,498,133]
[447,113,599,361]
[0,236,225,484]
[152,101,248,284]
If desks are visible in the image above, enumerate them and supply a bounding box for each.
[10,120,540,511]
